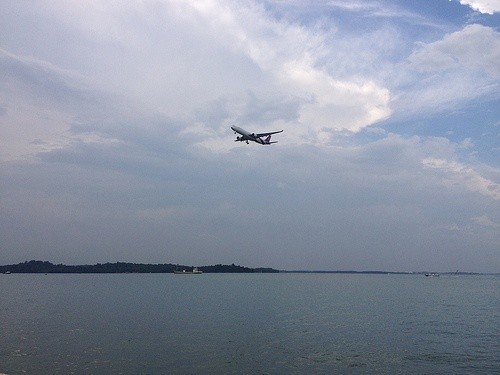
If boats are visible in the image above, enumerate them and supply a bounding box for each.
[174,270,203,274]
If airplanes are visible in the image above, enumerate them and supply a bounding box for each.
[230,124,284,146]
